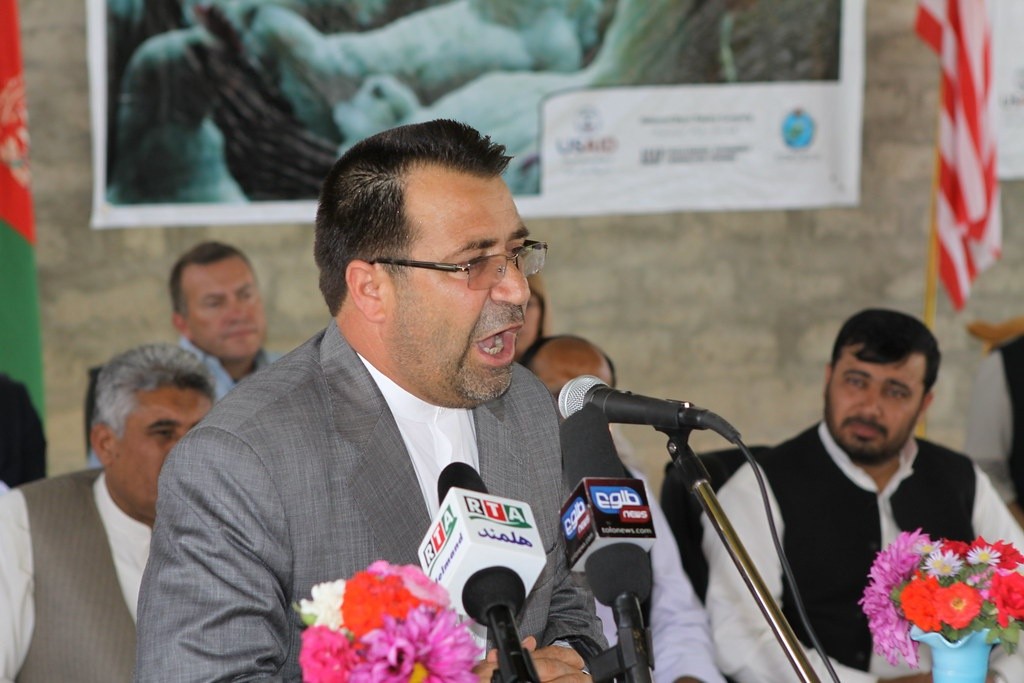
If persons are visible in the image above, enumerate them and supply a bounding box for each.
[516,270,729,683]
[169,240,284,409]
[0,343,217,683]
[695,308,1024,683]
[133,118,612,683]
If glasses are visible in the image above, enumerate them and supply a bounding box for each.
[370,239,549,291]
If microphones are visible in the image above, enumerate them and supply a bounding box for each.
[559,402,658,683]
[558,374,740,444]
[417,462,547,683]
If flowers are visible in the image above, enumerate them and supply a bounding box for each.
[857,528,1024,671]
[292,560,485,683]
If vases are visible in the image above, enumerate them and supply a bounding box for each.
[910,624,1013,683]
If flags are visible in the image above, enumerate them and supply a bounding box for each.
[916,0,1003,312]
[0,1,46,428]
